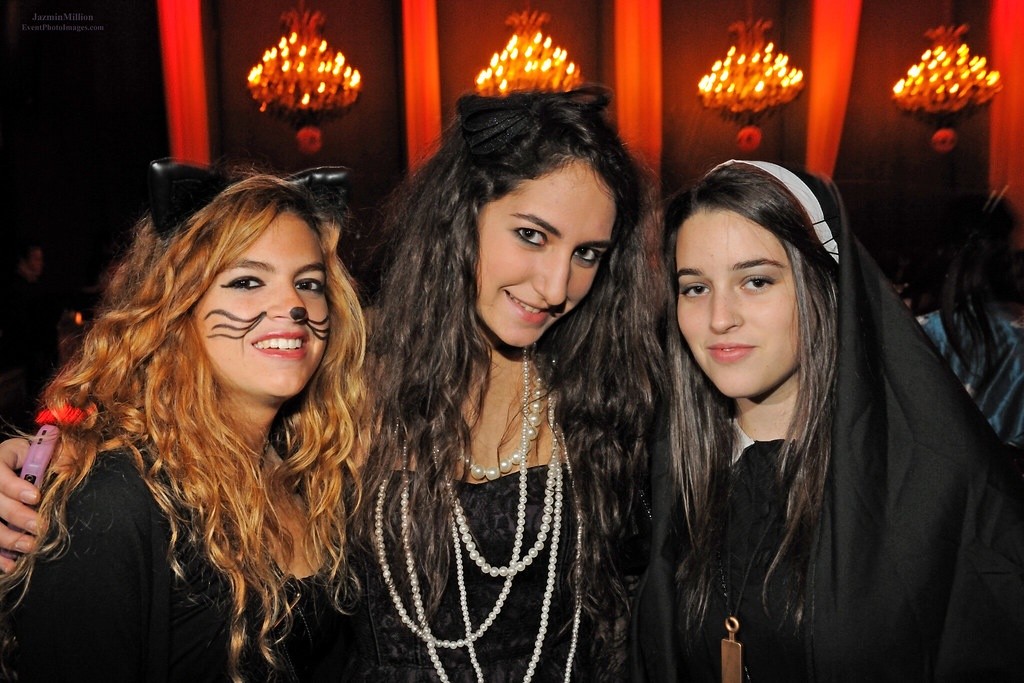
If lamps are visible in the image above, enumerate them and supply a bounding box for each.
[695,0,806,152]
[474,0,582,98]
[890,0,1005,153]
[246,0,366,156]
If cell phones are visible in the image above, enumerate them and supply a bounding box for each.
[0,423,60,560]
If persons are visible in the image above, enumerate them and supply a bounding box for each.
[0,85,654,683]
[918,198,1024,310]
[0,159,382,683]
[0,246,104,365]
[633,160,1024,683]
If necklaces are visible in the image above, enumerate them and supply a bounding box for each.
[716,512,778,683]
[374,346,582,683]
